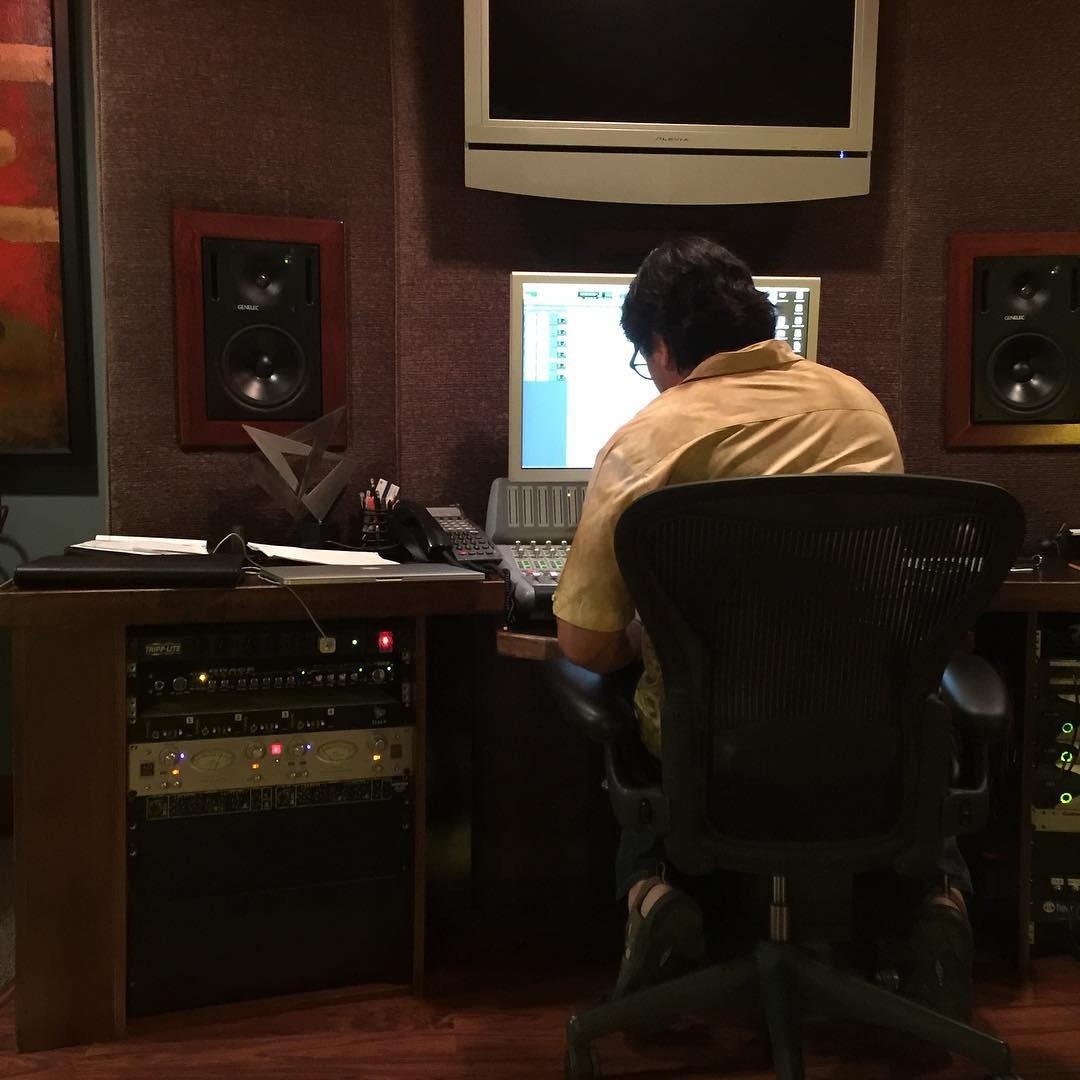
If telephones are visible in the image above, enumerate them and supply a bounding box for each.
[405,503,503,569]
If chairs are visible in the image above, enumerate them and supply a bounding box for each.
[549,474,1022,1080]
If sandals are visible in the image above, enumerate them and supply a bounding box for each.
[909,888,974,1027]
[619,878,704,1039]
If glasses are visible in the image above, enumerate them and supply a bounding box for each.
[631,342,654,379]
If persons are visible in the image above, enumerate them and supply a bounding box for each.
[552,237,977,1003]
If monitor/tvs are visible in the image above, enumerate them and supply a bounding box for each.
[463,0,881,206]
[507,271,822,483]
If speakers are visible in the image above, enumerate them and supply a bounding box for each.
[202,235,323,424]
[971,255,1080,423]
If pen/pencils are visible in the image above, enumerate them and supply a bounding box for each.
[361,478,400,542]
